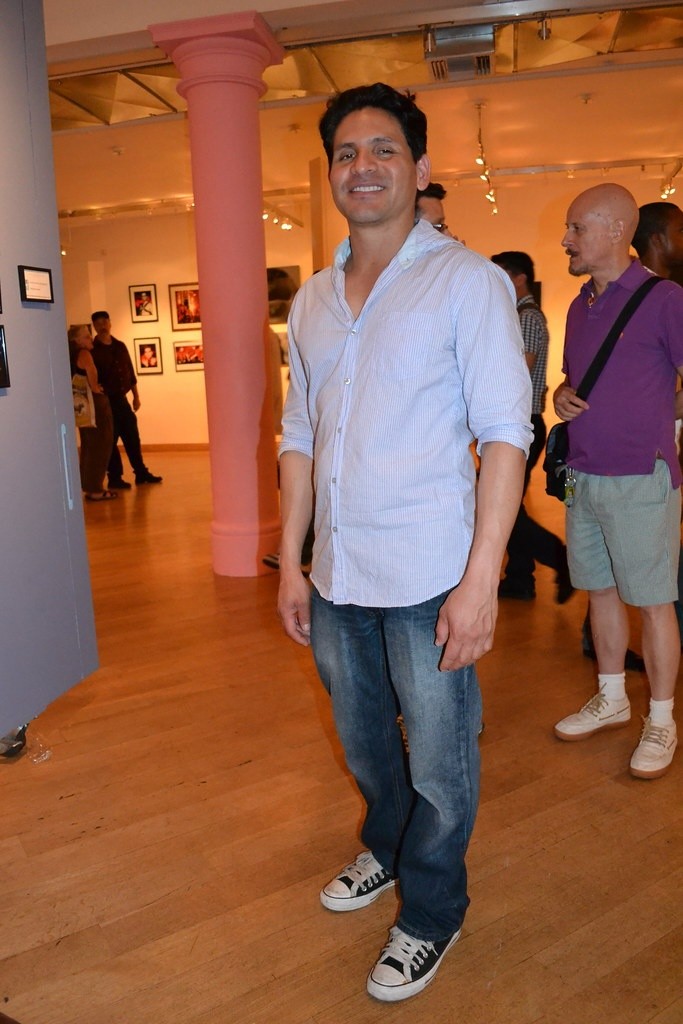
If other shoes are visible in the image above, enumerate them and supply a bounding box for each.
[263,552,311,579]
[584,645,646,673]
[107,480,131,489]
[498,580,537,601]
[555,581,576,605]
[135,473,162,484]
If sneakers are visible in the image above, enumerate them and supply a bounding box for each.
[366,925,462,1000]
[320,850,399,911]
[629,714,678,779]
[553,681,632,741]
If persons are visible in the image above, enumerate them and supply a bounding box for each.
[91,311,163,489]
[395,183,448,750]
[139,292,152,315]
[264,270,322,569]
[68,325,117,501]
[141,346,156,366]
[581,202,683,673]
[554,184,683,778]
[277,83,533,1000]
[493,251,578,603]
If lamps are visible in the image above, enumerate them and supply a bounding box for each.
[660,177,675,199]
[475,102,497,215]
[262,208,292,230]
[535,12,552,41]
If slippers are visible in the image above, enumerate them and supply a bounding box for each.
[85,490,118,501]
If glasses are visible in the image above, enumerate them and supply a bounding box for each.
[432,223,448,232]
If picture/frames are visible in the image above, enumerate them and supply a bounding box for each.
[128,285,159,323]
[134,337,163,375]
[169,282,202,332]
[174,340,205,372]
[18,265,54,303]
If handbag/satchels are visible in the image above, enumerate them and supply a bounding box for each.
[72,375,97,428]
[542,422,569,501]
